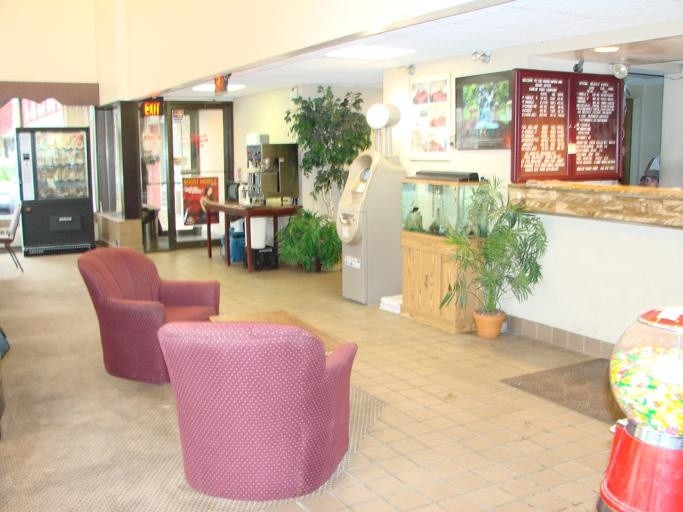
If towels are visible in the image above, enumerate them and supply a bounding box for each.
[140,207,161,252]
[221,231,246,263]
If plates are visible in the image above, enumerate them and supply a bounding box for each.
[15,127,97,258]
[401,227,489,335]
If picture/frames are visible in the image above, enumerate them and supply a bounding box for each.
[205,199,304,273]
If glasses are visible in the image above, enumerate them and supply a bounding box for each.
[614,63,630,79]
[470,51,492,66]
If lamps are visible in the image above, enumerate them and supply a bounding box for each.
[0,199,23,274]
[156,320,359,501]
[77,247,221,386]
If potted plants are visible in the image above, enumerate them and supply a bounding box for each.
[454,68,516,149]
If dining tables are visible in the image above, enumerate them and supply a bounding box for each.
[437,175,551,340]
[273,208,343,274]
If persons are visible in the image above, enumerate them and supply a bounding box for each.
[197,184,216,223]
